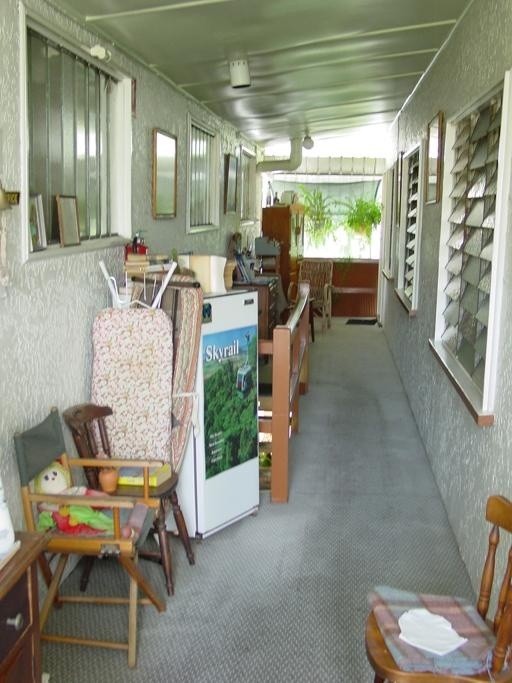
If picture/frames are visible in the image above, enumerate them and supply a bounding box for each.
[28,192,80,252]
[224,154,238,215]
[153,128,178,219]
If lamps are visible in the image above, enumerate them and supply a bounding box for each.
[229,60,251,88]
[302,134,313,150]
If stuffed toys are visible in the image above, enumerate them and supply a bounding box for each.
[35,462,135,538]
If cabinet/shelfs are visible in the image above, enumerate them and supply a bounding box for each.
[0,529,52,682]
[231,275,280,363]
[254,205,302,301]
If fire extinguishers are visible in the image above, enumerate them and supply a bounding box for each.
[123,228,148,287]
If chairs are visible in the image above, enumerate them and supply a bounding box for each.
[363,495,512,683]
[288,258,335,336]
[12,409,167,668]
[64,404,197,595]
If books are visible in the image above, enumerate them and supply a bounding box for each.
[113,464,171,487]
[125,255,170,274]
